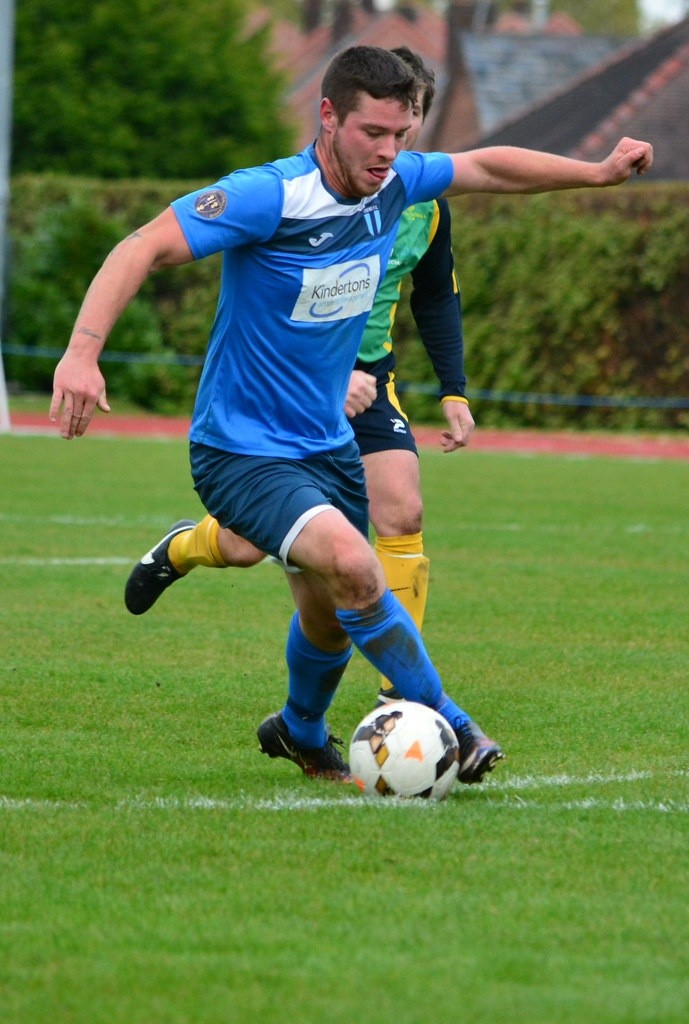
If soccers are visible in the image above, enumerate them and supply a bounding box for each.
[348,701,461,804]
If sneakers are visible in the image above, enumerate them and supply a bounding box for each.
[375,686,404,709]
[124,518,199,615]
[449,714,505,785]
[255,712,351,781]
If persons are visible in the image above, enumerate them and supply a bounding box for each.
[48,42,654,785]
[126,48,474,707]
